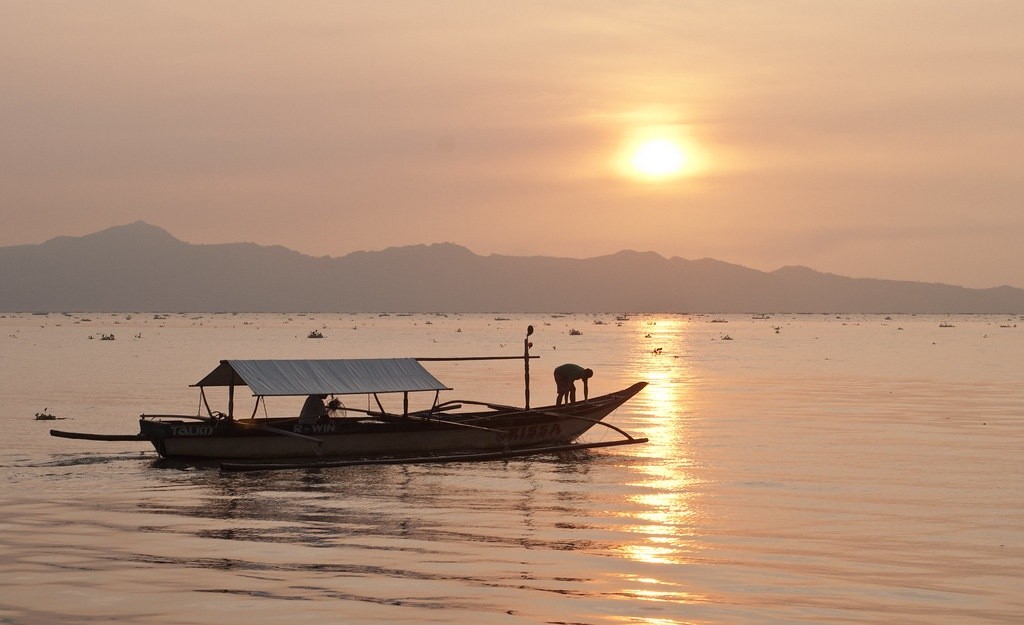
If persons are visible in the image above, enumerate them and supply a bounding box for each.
[554,363,593,405]
[297,394,331,424]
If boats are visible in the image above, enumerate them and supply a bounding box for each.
[138,356,648,465]
